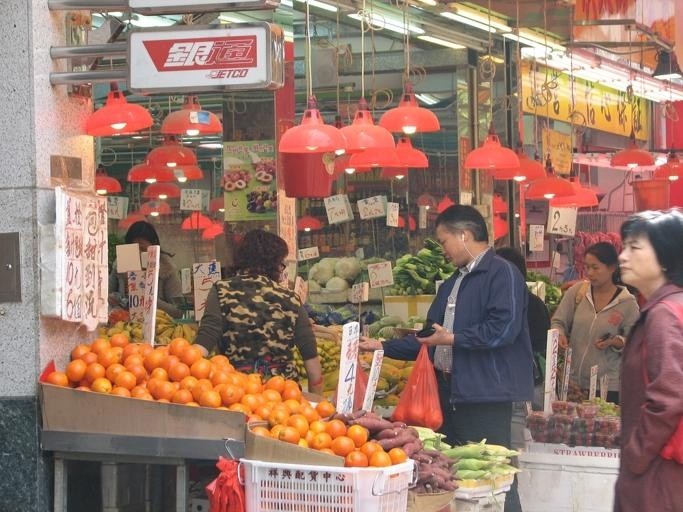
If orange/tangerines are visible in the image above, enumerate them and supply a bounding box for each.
[47,334,401,512]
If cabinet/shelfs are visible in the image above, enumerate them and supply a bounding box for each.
[39,381,245,512]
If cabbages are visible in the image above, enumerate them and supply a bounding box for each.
[306,259,359,293]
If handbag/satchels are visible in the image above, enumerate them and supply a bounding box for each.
[640,300,683,463]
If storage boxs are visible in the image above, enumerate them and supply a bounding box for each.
[237,421,415,512]
[407,490,455,512]
[516,443,622,512]
[384,294,435,321]
[453,477,514,512]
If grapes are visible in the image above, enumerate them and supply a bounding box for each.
[529,396,628,447]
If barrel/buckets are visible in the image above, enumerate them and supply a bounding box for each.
[281,152,333,198]
[629,178,670,212]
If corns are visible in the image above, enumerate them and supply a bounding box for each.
[410,426,451,451]
[449,437,523,478]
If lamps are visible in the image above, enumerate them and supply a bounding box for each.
[493,215,508,240]
[436,127,456,214]
[296,199,325,231]
[182,212,210,230]
[651,49,683,80]
[348,1,397,169]
[128,95,174,184]
[389,1,425,168]
[161,14,224,135]
[95,174,123,195]
[654,52,683,181]
[494,0,547,181]
[142,181,181,199]
[118,211,146,229]
[334,46,374,174]
[381,167,408,180]
[609,27,655,167]
[146,93,198,166]
[173,164,205,183]
[525,0,577,200]
[202,223,223,240]
[549,0,598,208]
[493,192,507,213]
[415,132,437,208]
[85,11,154,137]
[140,200,170,217]
[338,1,396,152]
[462,0,520,169]
[379,0,440,132]
[209,196,223,214]
[279,1,348,154]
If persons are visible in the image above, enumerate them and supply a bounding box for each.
[108,222,184,318]
[195,229,323,394]
[552,241,639,403]
[495,247,551,408]
[612,209,683,508]
[360,204,529,507]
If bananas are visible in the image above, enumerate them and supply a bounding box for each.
[100,308,196,345]
[291,336,341,377]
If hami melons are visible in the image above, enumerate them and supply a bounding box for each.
[362,317,405,340]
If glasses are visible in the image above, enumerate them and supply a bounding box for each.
[278,261,286,271]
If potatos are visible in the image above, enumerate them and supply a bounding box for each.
[322,410,463,493]
